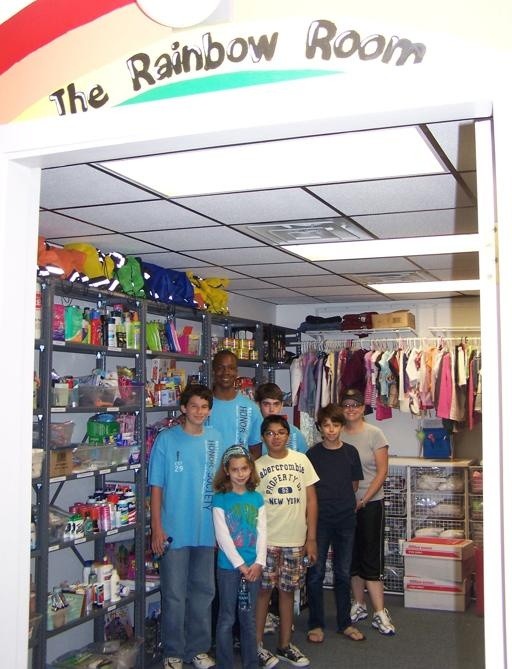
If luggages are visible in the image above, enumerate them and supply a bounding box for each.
[341,311,377,329]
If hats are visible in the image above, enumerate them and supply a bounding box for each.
[342,388,363,404]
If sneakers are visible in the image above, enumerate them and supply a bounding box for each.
[190,652,216,668]
[232,612,280,649]
[257,648,279,668]
[349,597,368,623]
[163,656,183,668]
[275,641,310,666]
[371,607,396,635]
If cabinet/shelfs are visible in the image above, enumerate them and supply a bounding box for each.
[31,275,298,668]
[378,456,482,598]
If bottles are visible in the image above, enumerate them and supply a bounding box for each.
[53,555,125,613]
[303,556,315,569]
[64,483,137,540]
[144,360,209,407]
[239,572,251,614]
[65,302,143,353]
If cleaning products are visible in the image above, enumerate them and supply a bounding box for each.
[64,486,137,543]
[154,383,169,406]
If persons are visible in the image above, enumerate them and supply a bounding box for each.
[249,417,320,668]
[213,445,265,668]
[305,404,369,641]
[147,383,228,669]
[253,381,311,455]
[334,389,394,634]
[206,351,261,459]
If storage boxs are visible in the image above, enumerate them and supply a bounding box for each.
[371,309,416,330]
[400,536,472,582]
[403,576,473,613]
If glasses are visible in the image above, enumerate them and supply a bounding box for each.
[265,430,285,437]
[344,403,360,409]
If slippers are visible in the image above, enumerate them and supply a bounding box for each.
[306,627,324,642]
[342,626,366,641]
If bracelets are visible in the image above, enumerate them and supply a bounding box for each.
[306,536,318,543]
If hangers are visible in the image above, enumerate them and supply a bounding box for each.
[300,337,480,358]
